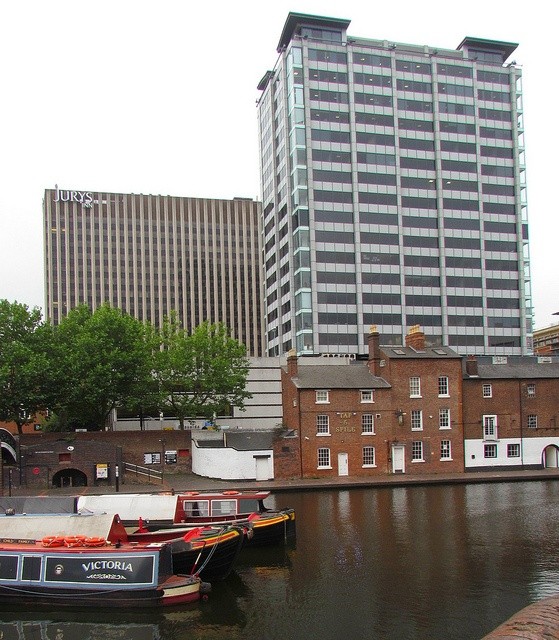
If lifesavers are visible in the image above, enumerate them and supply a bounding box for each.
[223,491,238,495]
[42,536,64,547]
[85,537,105,547]
[184,492,199,495]
[63,536,87,547]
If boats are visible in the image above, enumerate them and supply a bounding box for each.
[73,490,295,549]
[0,536,211,608]
[0,514,240,571]
